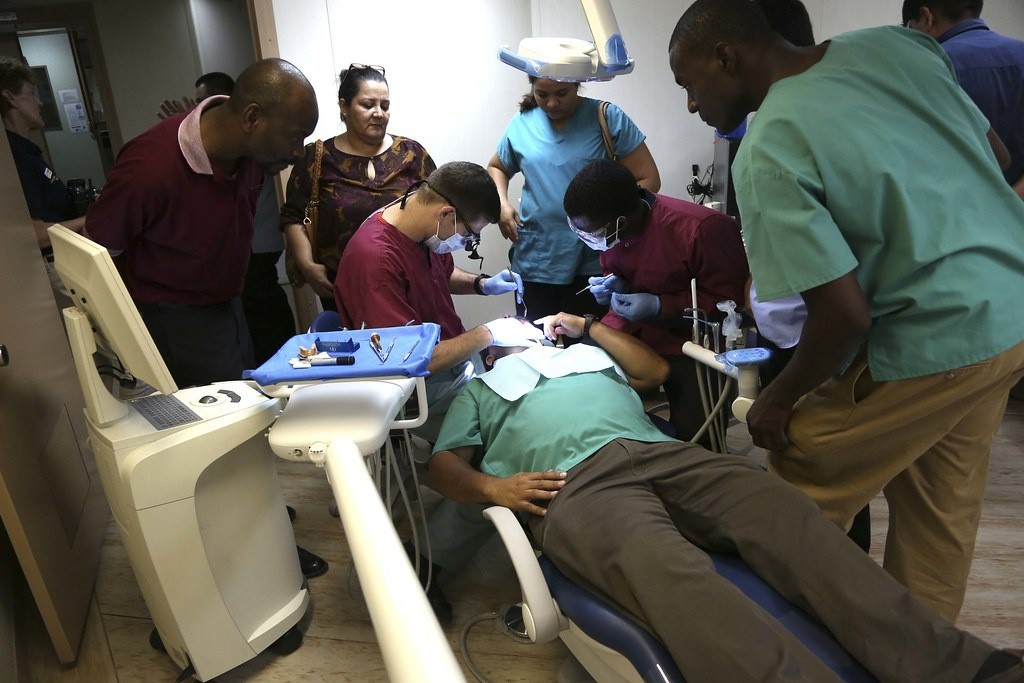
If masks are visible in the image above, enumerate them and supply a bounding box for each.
[580,216,626,252]
[423,208,474,255]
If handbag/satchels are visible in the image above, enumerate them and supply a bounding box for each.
[285,139,324,290]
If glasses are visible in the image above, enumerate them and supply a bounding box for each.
[452,205,481,241]
[567,215,615,239]
[341,63,385,83]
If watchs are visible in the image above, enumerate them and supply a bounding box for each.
[584,314,598,336]
[474,274,490,295]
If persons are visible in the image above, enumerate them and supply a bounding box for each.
[280,64,437,312]
[0,57,101,264]
[903,0,1024,200]
[563,160,753,452]
[487,71,661,349]
[334,161,525,625]
[428,314,1024,683]
[157,72,296,366]
[668,0,1024,625]
[85,57,329,581]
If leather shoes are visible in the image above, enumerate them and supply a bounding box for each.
[286,505,296,521]
[297,547,329,579]
